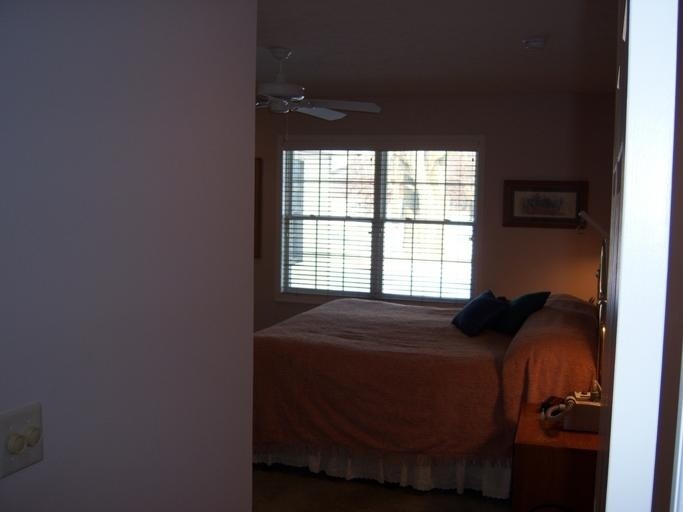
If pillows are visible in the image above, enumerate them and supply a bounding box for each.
[452,291,597,368]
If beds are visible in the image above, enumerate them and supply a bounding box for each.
[253,238,598,498]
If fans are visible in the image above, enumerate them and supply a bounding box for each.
[255,44,384,123]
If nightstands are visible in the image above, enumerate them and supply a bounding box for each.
[509,401,602,512]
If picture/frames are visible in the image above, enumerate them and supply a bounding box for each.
[502,178,589,229]
